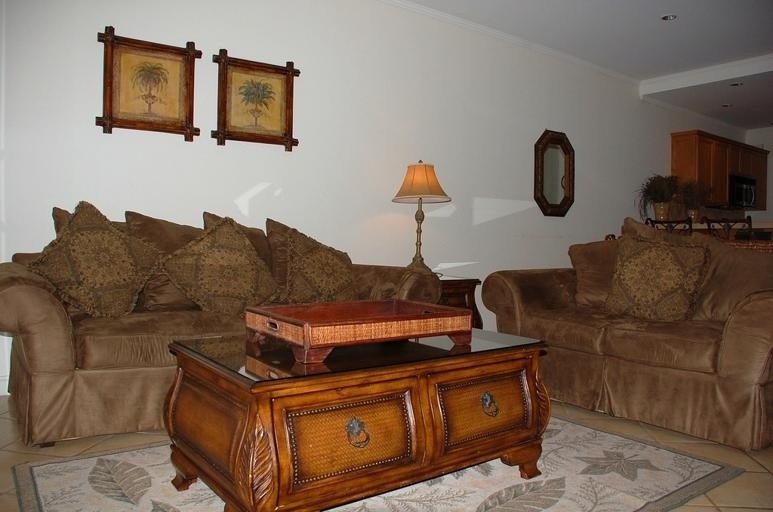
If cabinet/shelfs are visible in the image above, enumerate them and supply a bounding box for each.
[671,130,726,205]
[753,151,768,211]
[728,144,752,176]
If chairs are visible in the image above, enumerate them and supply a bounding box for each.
[700,216,753,240]
[645,217,693,232]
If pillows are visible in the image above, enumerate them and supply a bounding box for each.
[604,233,711,323]
[266,218,359,305]
[202,212,266,261]
[158,217,287,323]
[31,201,162,320]
[52,206,127,318]
[124,210,205,312]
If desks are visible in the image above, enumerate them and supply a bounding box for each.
[438,278,483,330]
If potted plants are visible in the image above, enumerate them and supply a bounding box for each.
[683,181,710,220]
[633,174,675,220]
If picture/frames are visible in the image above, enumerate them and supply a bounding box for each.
[211,48,299,152]
[95,25,202,142]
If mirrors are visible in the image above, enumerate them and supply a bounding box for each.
[533,129,575,217]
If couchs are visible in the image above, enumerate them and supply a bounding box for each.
[0,252,442,448]
[480,239,773,452]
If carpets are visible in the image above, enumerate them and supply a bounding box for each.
[12,417,748,511]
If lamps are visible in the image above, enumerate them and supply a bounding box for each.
[392,164,452,272]
